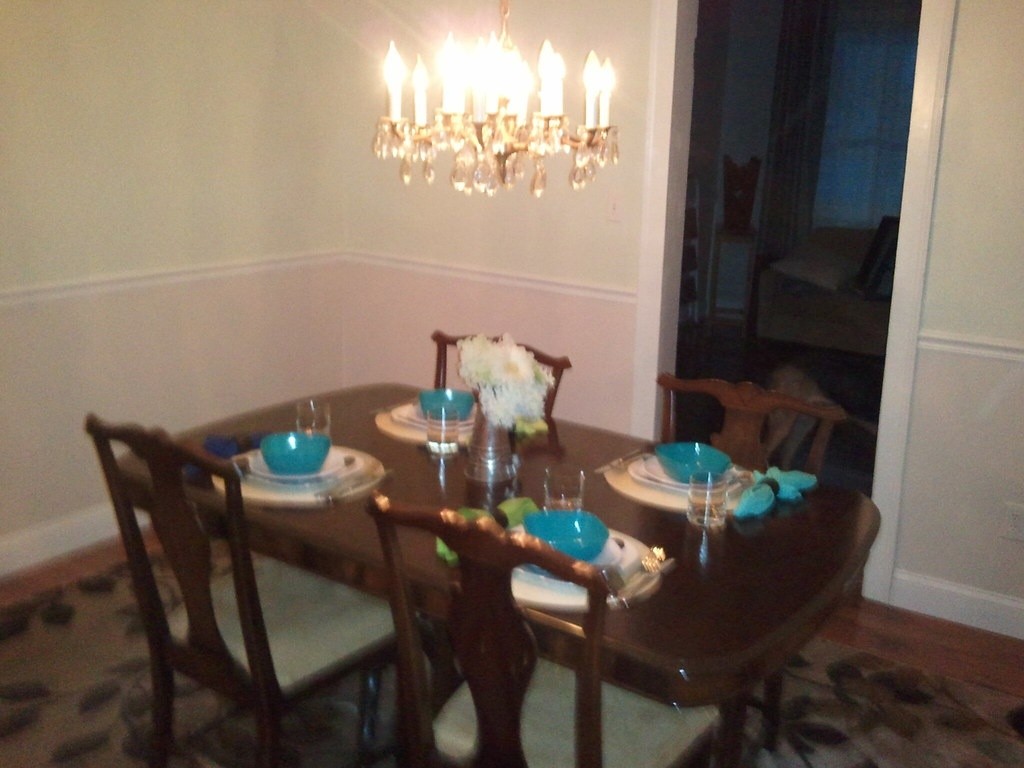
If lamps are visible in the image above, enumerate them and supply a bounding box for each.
[374,0,620,200]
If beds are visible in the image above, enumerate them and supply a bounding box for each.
[757,221,896,357]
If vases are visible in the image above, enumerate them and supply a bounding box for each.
[463,397,517,483]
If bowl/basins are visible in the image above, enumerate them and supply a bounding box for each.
[418,390,474,421]
[655,442,730,484]
[522,508,609,564]
[260,431,330,474]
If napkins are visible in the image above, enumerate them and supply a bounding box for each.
[181,425,276,478]
[735,463,817,519]
[510,416,550,435]
[436,494,540,562]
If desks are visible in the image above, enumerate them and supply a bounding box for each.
[116,381,883,768]
[710,228,759,341]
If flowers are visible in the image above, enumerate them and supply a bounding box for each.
[457,333,554,425]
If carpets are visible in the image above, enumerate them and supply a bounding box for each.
[1,537,1023,768]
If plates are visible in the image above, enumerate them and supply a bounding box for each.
[627,455,738,494]
[510,534,638,605]
[243,450,364,489]
[388,402,478,433]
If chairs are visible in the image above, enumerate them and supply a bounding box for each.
[84,330,847,768]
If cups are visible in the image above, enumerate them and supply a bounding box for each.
[542,465,585,513]
[426,408,459,464]
[686,471,728,529]
[295,397,330,437]
